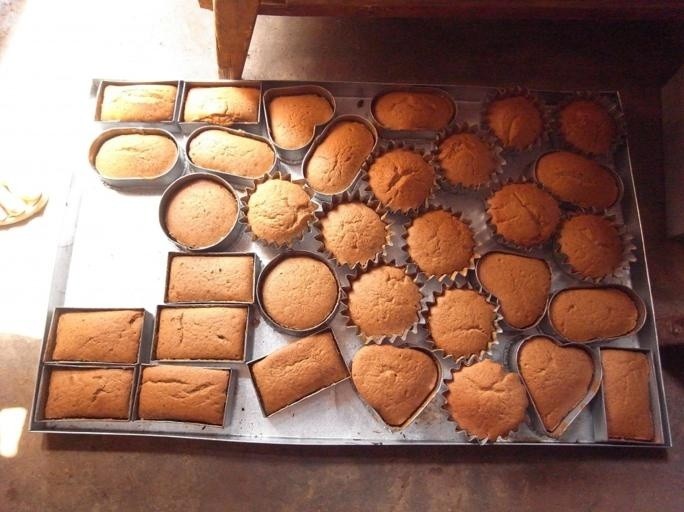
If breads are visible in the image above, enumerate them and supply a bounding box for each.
[517,336,594,432]
[156,307,249,360]
[319,200,388,269]
[167,255,255,303]
[486,182,562,248]
[600,349,656,441]
[305,119,375,195]
[99,84,177,123]
[45,368,134,419]
[262,255,338,330]
[183,86,261,125]
[367,147,436,214]
[557,97,618,158]
[95,132,178,181]
[556,212,625,279]
[478,252,552,329]
[427,288,497,361]
[406,210,474,278]
[351,343,438,427]
[374,90,455,131]
[444,358,530,443]
[267,92,334,150]
[164,178,237,249]
[437,131,498,189]
[251,330,349,415]
[536,151,619,210]
[51,310,144,364]
[347,264,423,339]
[247,178,315,247]
[486,94,543,150]
[549,287,640,342]
[187,128,275,180]
[138,366,230,425]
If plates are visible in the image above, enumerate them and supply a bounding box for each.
[31,78,673,450]
